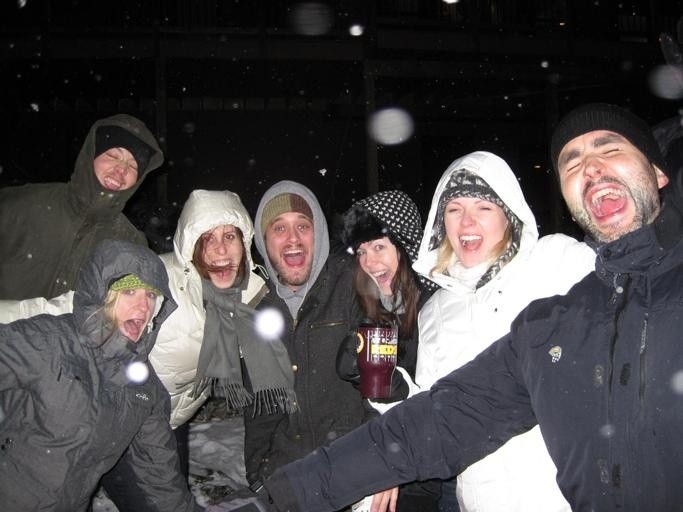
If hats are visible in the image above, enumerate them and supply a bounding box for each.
[261,194,314,235]
[348,215,388,241]
[549,100,672,181]
[111,274,162,297]
[95,125,151,183]
[437,169,522,234]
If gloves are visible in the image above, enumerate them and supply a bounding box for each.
[371,370,410,404]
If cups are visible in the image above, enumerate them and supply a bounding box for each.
[354,320,399,399]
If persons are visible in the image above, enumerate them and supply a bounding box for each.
[0,103,683,510]
